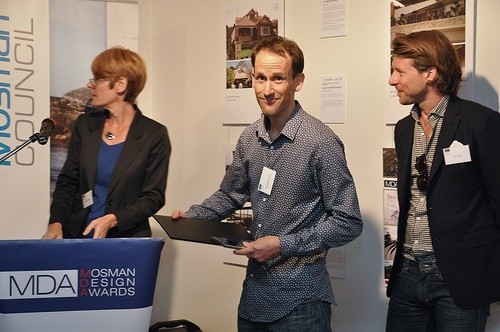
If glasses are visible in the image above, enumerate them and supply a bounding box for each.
[415,154,428,191]
[89,75,125,86]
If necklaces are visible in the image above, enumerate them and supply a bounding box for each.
[105,131,116,140]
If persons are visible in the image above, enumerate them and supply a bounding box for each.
[40,45,171,239]
[385,29,500,332]
[171,36,364,332]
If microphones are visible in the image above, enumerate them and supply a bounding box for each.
[38,117,56,145]
